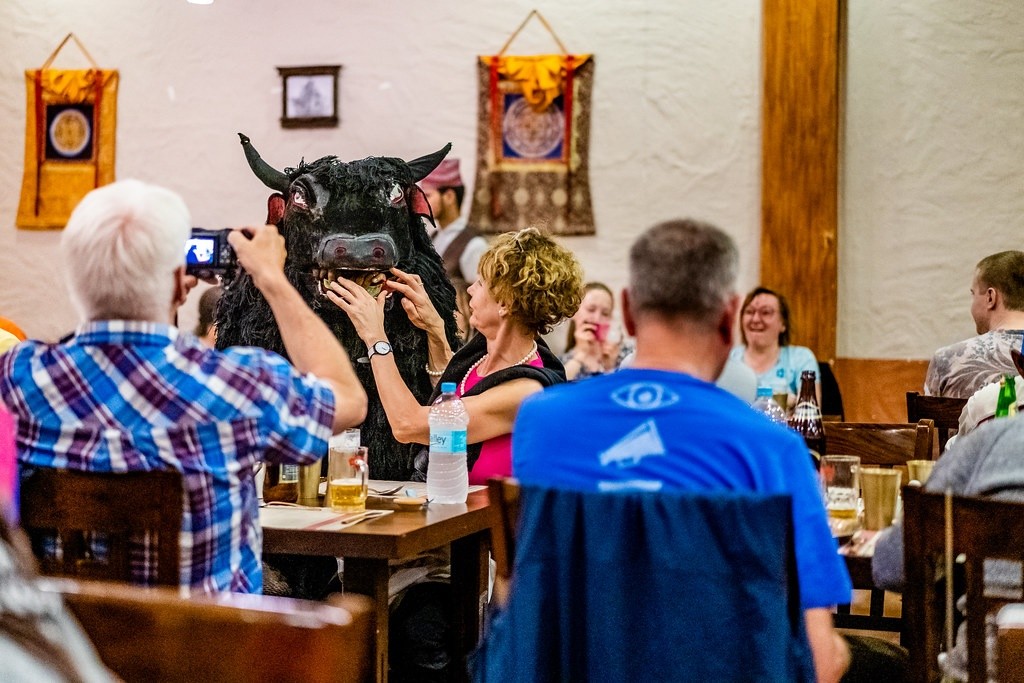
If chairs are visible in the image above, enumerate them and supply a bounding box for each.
[0,362,1024,683]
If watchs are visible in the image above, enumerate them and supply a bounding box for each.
[368,341,393,360]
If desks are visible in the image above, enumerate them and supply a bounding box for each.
[259,479,491,662]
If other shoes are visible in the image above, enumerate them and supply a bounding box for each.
[938,650,969,680]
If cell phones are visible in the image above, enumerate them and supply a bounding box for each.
[593,324,610,341]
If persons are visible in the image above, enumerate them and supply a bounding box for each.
[420,158,489,287]
[730,287,822,408]
[923,251,1024,399]
[872,407,1024,683]
[511,219,852,683]
[326,226,585,484]
[958,375,1024,439]
[556,282,634,381]
[0,180,368,595]
[195,286,227,349]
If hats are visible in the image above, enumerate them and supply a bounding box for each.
[421,159,462,187]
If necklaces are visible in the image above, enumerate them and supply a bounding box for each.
[461,340,538,397]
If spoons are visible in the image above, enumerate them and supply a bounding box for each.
[368,484,406,496]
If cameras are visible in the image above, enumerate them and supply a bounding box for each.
[185,228,250,278]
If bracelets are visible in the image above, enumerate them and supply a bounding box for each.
[426,351,455,376]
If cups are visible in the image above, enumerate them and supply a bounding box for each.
[329,427,361,484]
[327,446,370,512]
[297,457,321,507]
[773,392,787,410]
[860,468,903,529]
[819,455,862,519]
[907,460,936,485]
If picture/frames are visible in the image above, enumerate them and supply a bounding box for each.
[275,65,342,128]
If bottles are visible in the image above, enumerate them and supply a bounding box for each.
[749,387,789,427]
[789,370,827,470]
[428,382,468,505]
[995,373,1017,417]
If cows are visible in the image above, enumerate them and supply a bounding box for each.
[205,133,466,602]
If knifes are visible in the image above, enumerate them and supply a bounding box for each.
[342,512,383,524]
